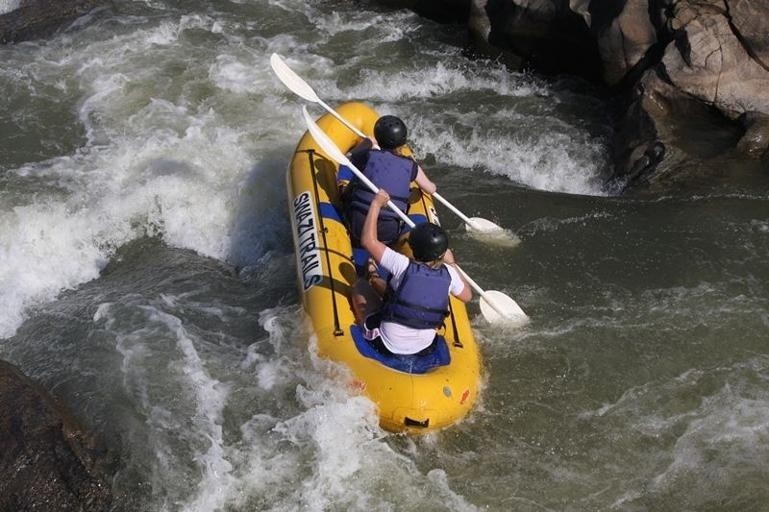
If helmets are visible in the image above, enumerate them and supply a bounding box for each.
[407,221,450,264]
[373,114,407,152]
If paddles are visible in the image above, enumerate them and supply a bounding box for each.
[271,53,521,248]
[302,104,529,326]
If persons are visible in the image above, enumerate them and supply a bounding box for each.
[336,115,437,246]
[349,187,473,357]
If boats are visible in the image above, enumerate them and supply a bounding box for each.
[285,101,482,437]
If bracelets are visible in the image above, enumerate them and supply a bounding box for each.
[447,261,456,265]
